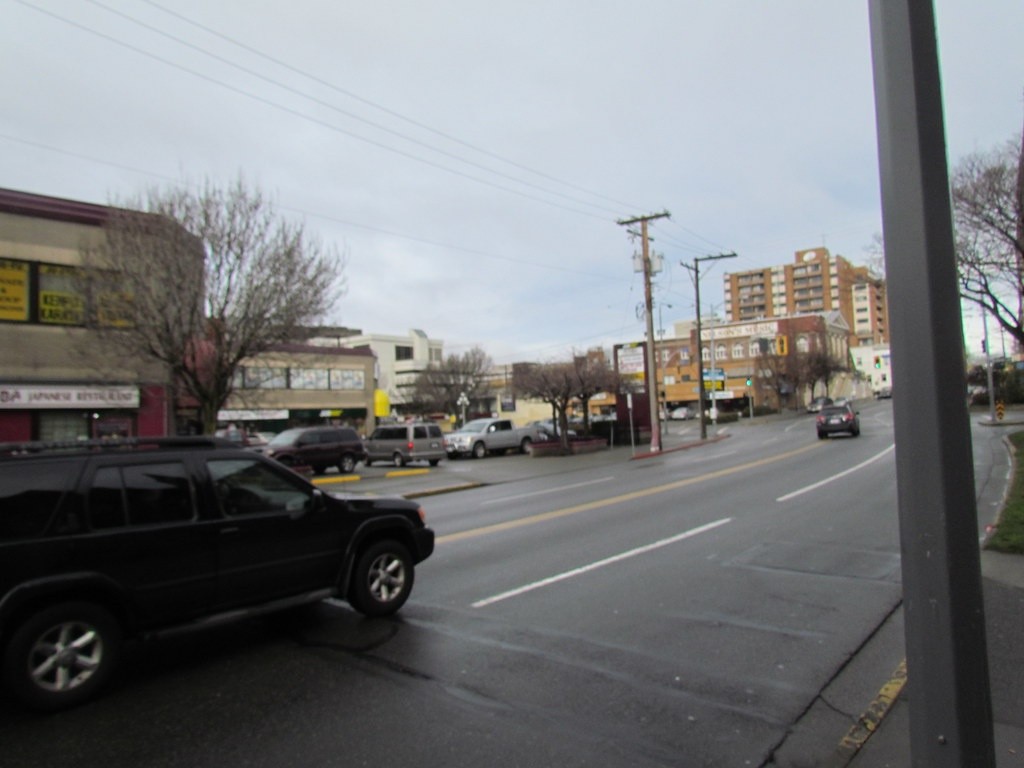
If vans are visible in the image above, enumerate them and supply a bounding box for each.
[264,426,366,474]
[363,423,447,469]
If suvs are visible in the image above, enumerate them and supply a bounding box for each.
[0,433,435,713]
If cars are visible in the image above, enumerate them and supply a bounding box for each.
[815,406,860,439]
[807,395,854,413]
[671,407,695,421]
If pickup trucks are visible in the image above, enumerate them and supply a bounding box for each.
[444,418,536,459]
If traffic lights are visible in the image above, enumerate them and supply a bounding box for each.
[746,375,752,386]
[875,357,881,368]
